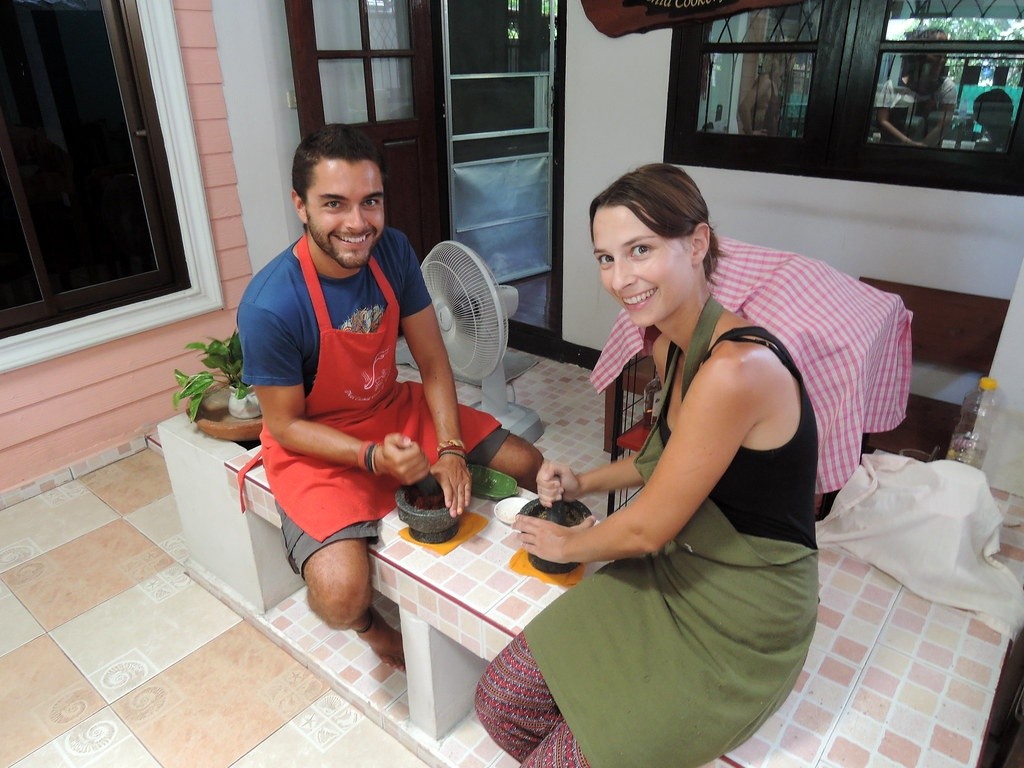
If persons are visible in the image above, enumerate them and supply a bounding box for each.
[875,28,958,148]
[736,31,796,135]
[973,88,1014,152]
[236,124,545,672]
[473,163,818,768]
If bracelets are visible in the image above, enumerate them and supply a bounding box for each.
[905,137,913,145]
[357,439,381,476]
[437,439,471,466]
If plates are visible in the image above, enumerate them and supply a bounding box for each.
[467,464,517,501]
[494,497,530,525]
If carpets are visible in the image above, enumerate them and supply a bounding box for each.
[396,336,539,388]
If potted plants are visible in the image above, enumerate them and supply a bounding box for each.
[172,327,262,424]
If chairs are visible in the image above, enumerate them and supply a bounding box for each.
[818,275,1011,521]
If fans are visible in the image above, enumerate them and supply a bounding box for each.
[419,240,545,445]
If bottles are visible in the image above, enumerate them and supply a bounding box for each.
[946,377,998,469]
[958,99,967,118]
